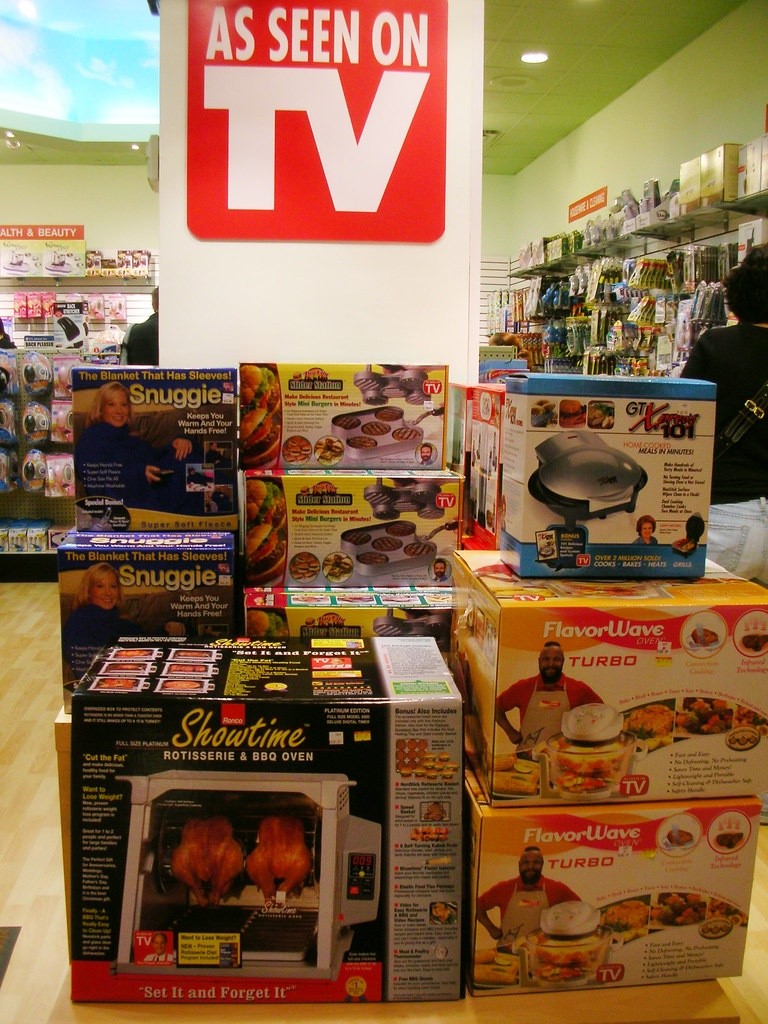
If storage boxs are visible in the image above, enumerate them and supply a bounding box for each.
[58,362,767,1004]
[622,136,768,237]
[12,250,151,321]
[0,519,76,552]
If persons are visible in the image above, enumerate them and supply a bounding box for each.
[479,846,584,952]
[75,381,229,514]
[632,515,659,545]
[494,642,605,761]
[680,244,768,590]
[63,564,185,676]
[127,286,160,366]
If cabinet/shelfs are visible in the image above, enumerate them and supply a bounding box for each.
[46,709,741,1023]
